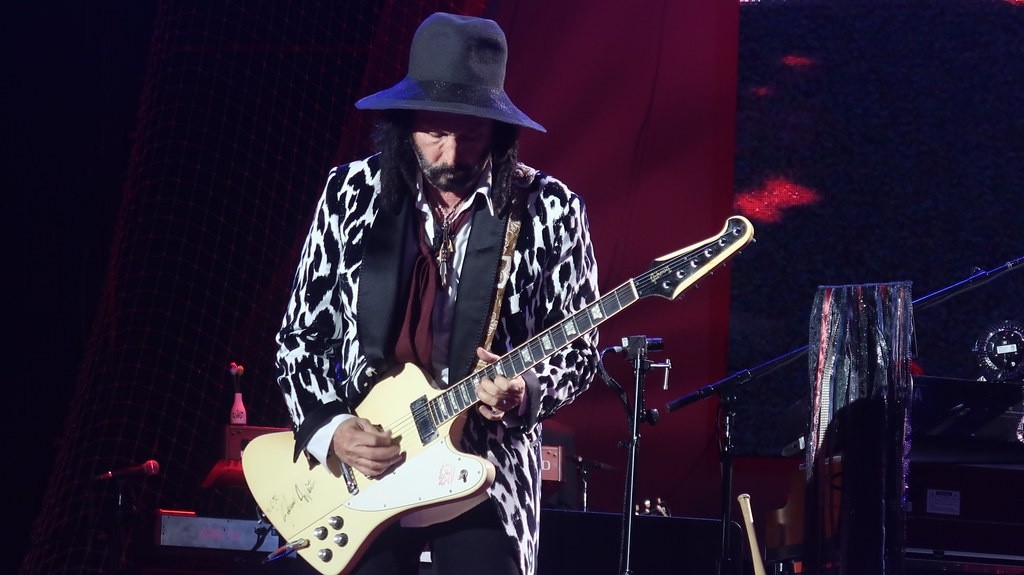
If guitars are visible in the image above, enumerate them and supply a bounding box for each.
[240,212,757,575]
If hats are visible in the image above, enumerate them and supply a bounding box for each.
[353,12,548,133]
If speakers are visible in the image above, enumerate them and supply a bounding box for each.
[900,368,1024,575]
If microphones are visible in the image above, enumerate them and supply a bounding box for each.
[102,460,160,481]
[645,407,659,424]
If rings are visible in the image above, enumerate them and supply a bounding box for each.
[365,475,371,478]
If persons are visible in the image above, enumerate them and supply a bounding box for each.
[273,12,601,575]
